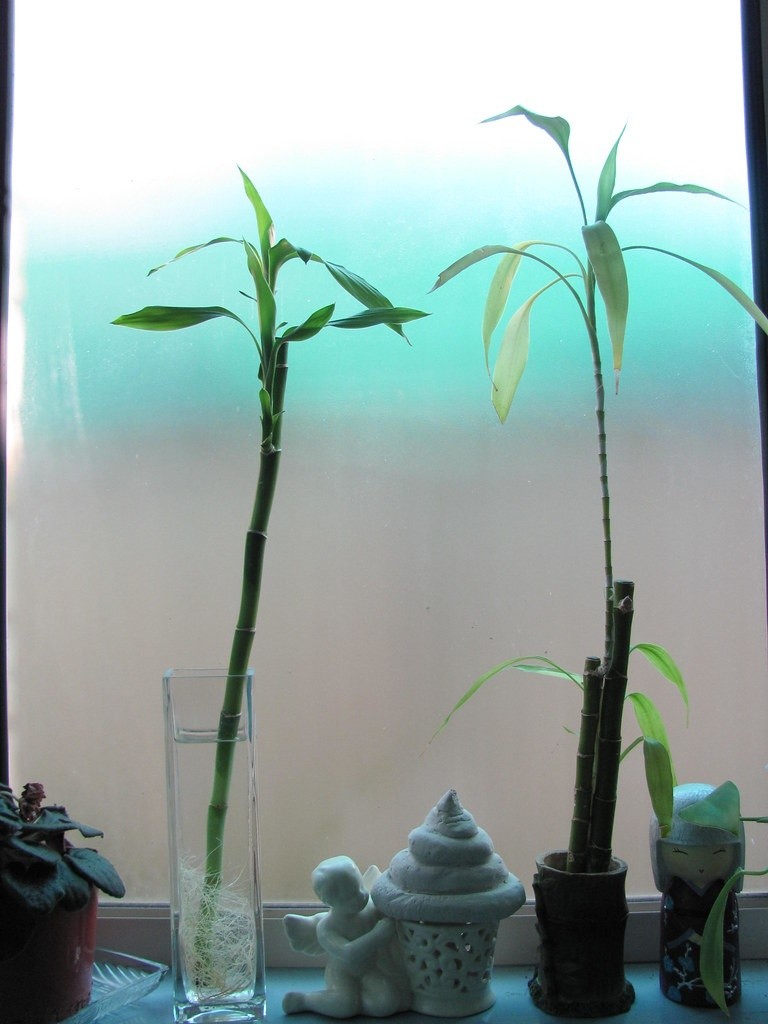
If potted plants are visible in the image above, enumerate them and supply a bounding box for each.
[427,105,767,1021]
[109,168,431,1024]
[0,779,129,1024]
